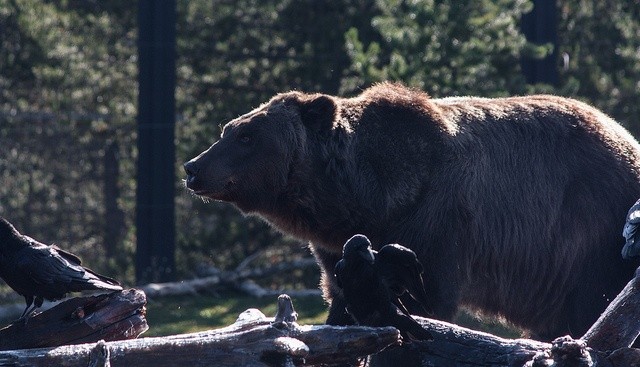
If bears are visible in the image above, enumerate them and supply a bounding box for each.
[183,76,640,345]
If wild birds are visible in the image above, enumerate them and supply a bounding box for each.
[333,231,435,343]
[0,215,124,329]
